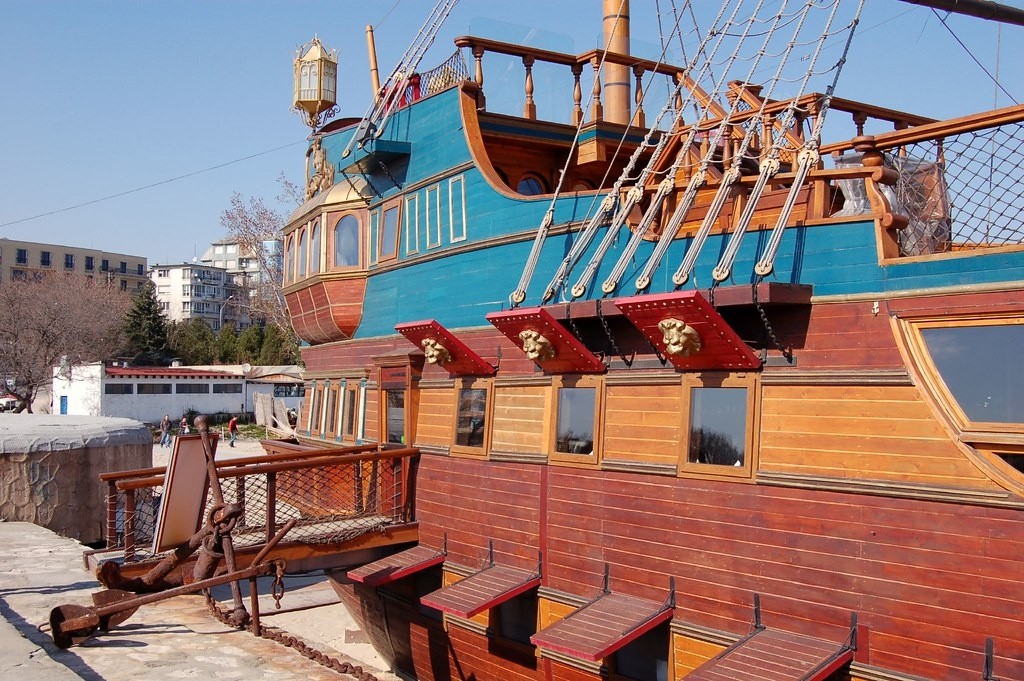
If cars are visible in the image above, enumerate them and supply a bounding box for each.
[0,397,17,413]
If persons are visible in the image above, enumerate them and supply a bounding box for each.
[228,415,238,447]
[159,415,172,448]
[178,418,187,435]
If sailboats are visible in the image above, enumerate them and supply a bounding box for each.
[259,0,1023,681]
